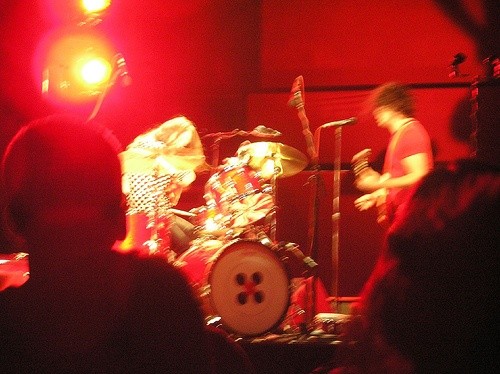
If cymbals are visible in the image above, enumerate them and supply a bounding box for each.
[237,139,309,179]
[113,145,205,175]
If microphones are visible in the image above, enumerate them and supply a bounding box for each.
[117,58,131,86]
[450,53,465,67]
[255,125,281,135]
[322,117,357,129]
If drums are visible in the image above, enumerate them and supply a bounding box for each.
[175,238,293,338]
[203,163,274,229]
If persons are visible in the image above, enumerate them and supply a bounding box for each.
[351,81,434,308]
[119,116,212,255]
[0,117,256,374]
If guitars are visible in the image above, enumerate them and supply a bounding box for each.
[346,145,391,229]
